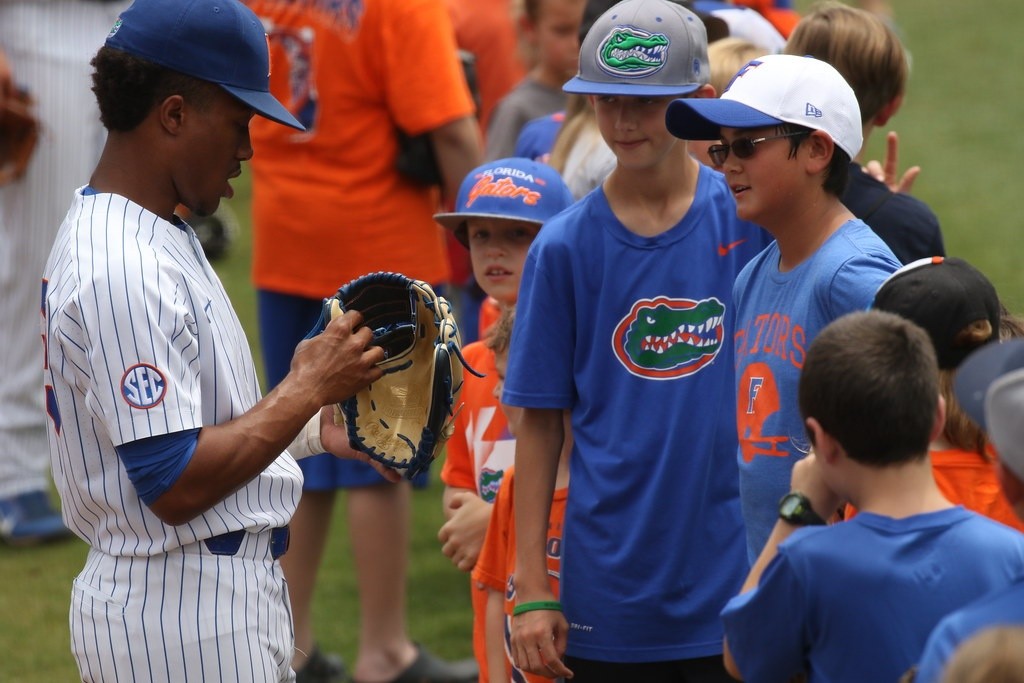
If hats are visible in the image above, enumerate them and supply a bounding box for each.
[513,112,565,161]
[955,335,1023,478]
[665,54,863,162]
[875,256,999,365]
[704,7,791,56]
[105,0,306,130]
[434,157,573,223]
[561,0,710,95]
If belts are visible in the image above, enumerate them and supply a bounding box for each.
[204,525,291,558]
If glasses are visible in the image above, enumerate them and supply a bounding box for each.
[707,130,810,165]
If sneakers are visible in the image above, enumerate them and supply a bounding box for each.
[0,491,72,545]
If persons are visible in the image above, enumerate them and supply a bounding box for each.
[900,339,1024,683]
[0,0,136,543]
[720,310,1024,683]
[666,56,901,567]
[872,255,1024,533]
[673,0,946,265]
[42,0,403,683]
[244,0,575,683]
[502,0,776,683]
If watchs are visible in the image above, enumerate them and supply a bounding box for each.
[779,492,827,526]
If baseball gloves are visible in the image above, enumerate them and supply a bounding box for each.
[307,274,465,468]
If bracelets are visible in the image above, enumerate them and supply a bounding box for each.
[512,600,563,615]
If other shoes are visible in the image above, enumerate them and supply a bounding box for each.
[347,638,480,683]
[293,642,349,682]
